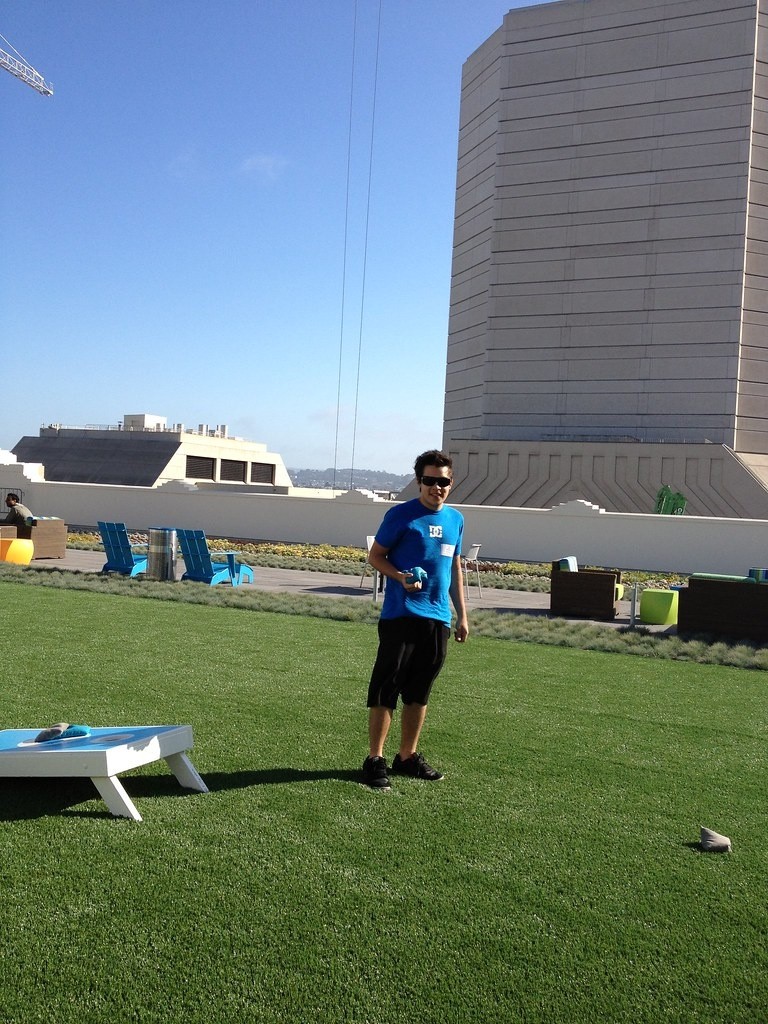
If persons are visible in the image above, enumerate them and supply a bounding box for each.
[362,450,470,789]
[0,493,34,534]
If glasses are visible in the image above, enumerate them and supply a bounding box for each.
[420,476,451,487]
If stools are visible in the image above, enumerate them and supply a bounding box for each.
[0,538,35,565]
[640,588,678,626]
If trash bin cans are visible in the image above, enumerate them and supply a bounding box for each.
[145,528,177,580]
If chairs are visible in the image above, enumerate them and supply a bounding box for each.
[547,555,623,620]
[358,535,388,593]
[0,516,68,561]
[0,725,208,821]
[670,566,768,643]
[462,544,483,601]
[97,520,149,577]
[174,528,256,587]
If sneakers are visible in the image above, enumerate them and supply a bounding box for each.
[392,752,444,782]
[363,755,389,786]
